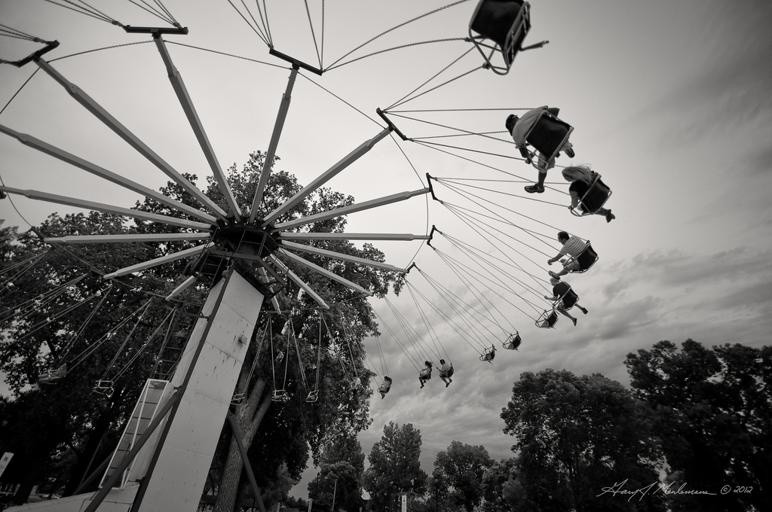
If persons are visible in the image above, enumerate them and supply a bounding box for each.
[38,106,615,400]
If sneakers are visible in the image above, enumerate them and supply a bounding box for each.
[581,307,588,315]
[381,394,385,399]
[606,209,616,223]
[573,318,577,326]
[566,148,576,158]
[549,270,560,281]
[445,379,452,387]
[524,184,545,194]
[420,380,426,388]
